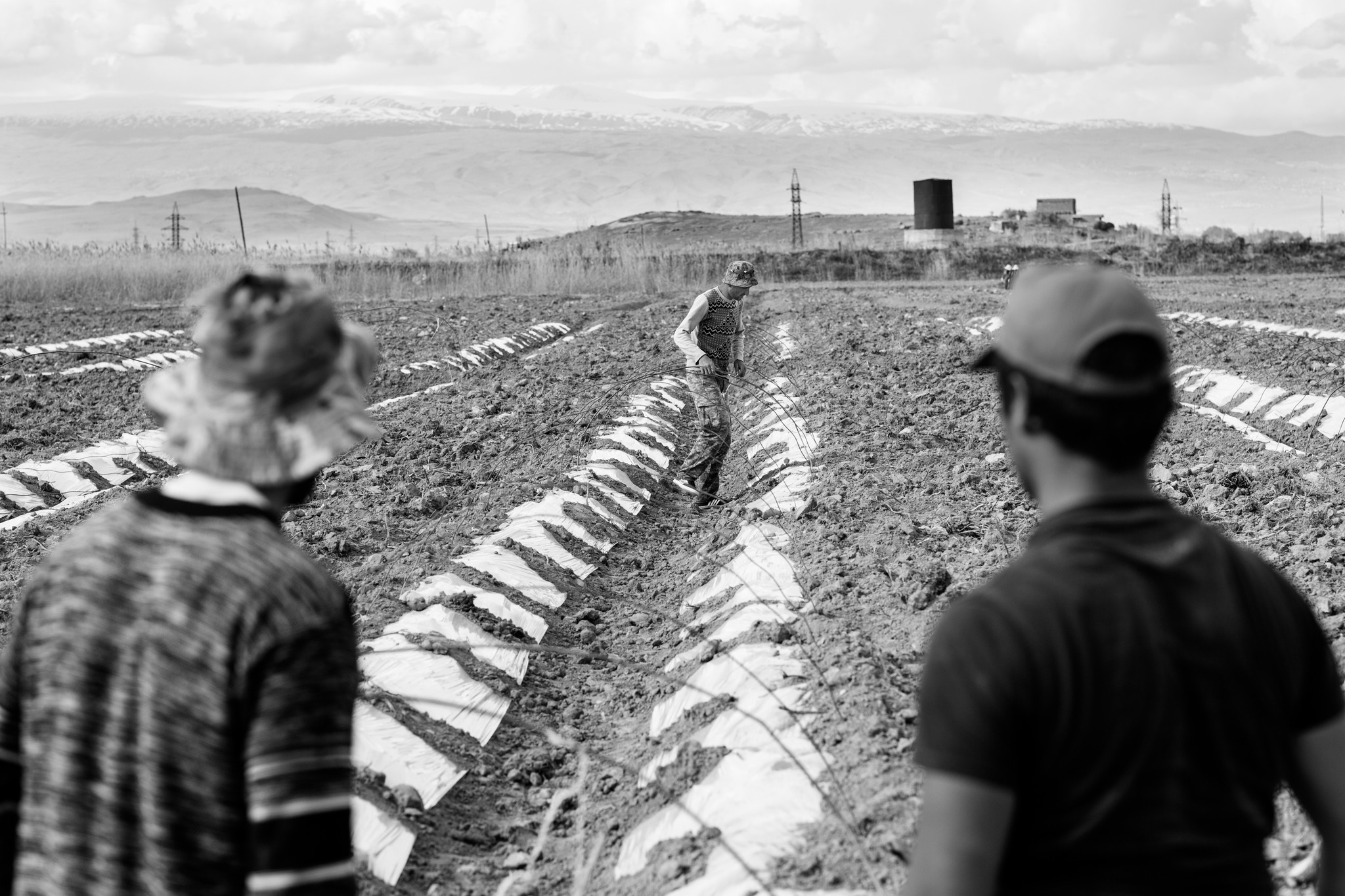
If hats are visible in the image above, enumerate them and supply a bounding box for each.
[966,258,1178,390]
[1012,265,1019,270]
[142,265,383,488]
[722,260,759,289]
[1005,264,1011,270]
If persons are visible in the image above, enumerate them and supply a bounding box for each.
[0,263,376,896]
[889,258,1345,896]
[671,261,760,512]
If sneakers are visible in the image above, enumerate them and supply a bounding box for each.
[695,497,734,509]
[673,477,699,497]
